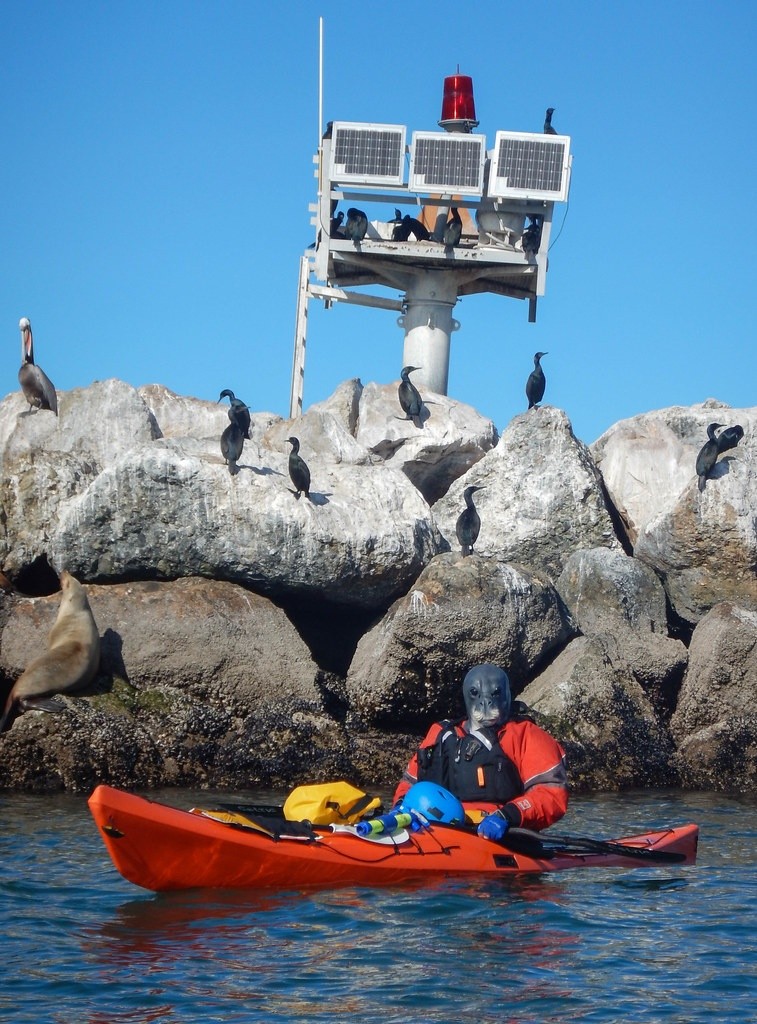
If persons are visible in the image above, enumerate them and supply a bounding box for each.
[393,664,570,842]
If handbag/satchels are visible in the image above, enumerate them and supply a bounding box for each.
[281,781,381,828]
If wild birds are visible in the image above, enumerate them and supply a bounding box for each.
[394,366,424,429]
[456,486,487,559]
[316,108,559,262]
[19,317,59,416]
[217,389,251,476]
[527,352,549,409]
[694,423,744,493]
[284,437,310,497]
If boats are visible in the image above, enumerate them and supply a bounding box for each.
[87,780,701,896]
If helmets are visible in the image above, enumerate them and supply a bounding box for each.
[399,780,465,828]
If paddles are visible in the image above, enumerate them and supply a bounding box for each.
[214,801,687,866]
